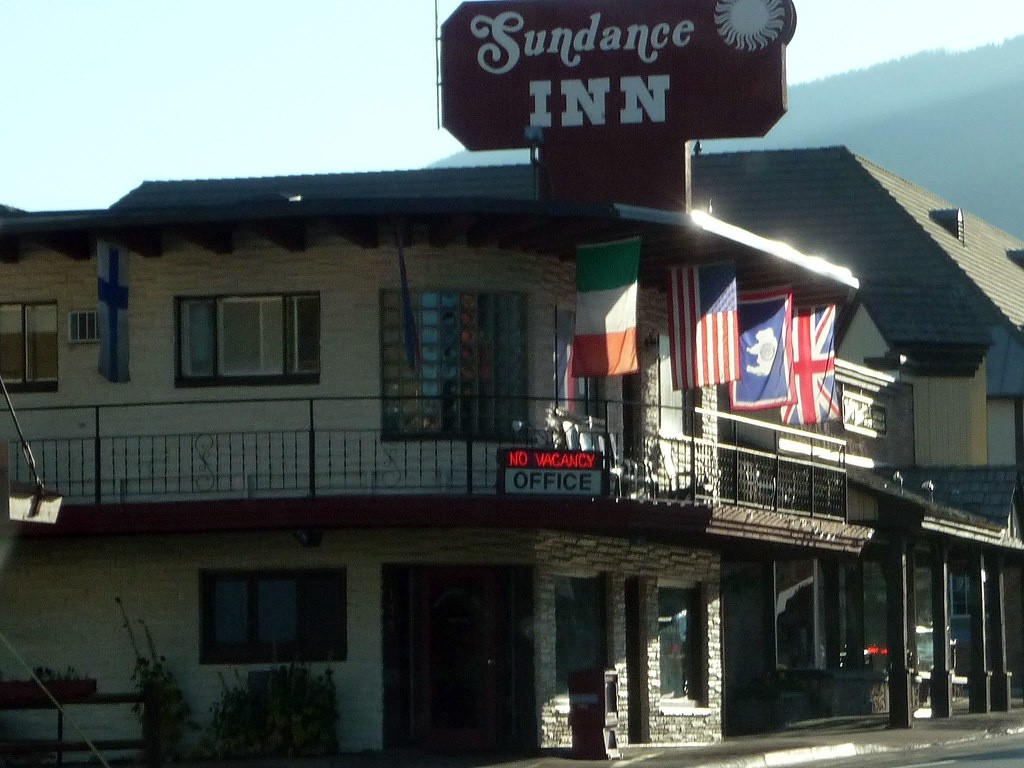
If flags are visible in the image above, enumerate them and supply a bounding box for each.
[662,255,743,391]
[397,236,420,372]
[569,234,643,377]
[779,301,842,425]
[95,235,131,383]
[728,283,798,411]
